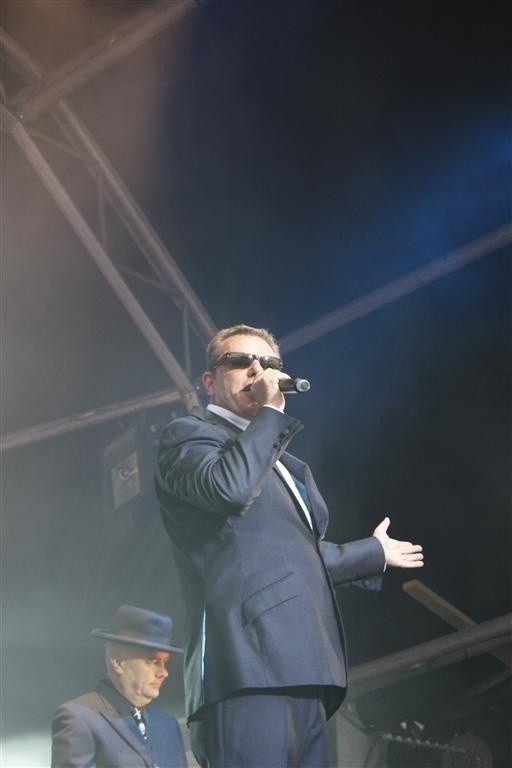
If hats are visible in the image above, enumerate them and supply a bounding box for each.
[92,603,185,658]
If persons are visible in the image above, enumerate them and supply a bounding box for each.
[153,322,424,766]
[50,606,190,767]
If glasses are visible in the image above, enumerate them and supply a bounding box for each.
[211,347,284,373]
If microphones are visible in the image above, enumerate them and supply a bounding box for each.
[279,378,311,393]
